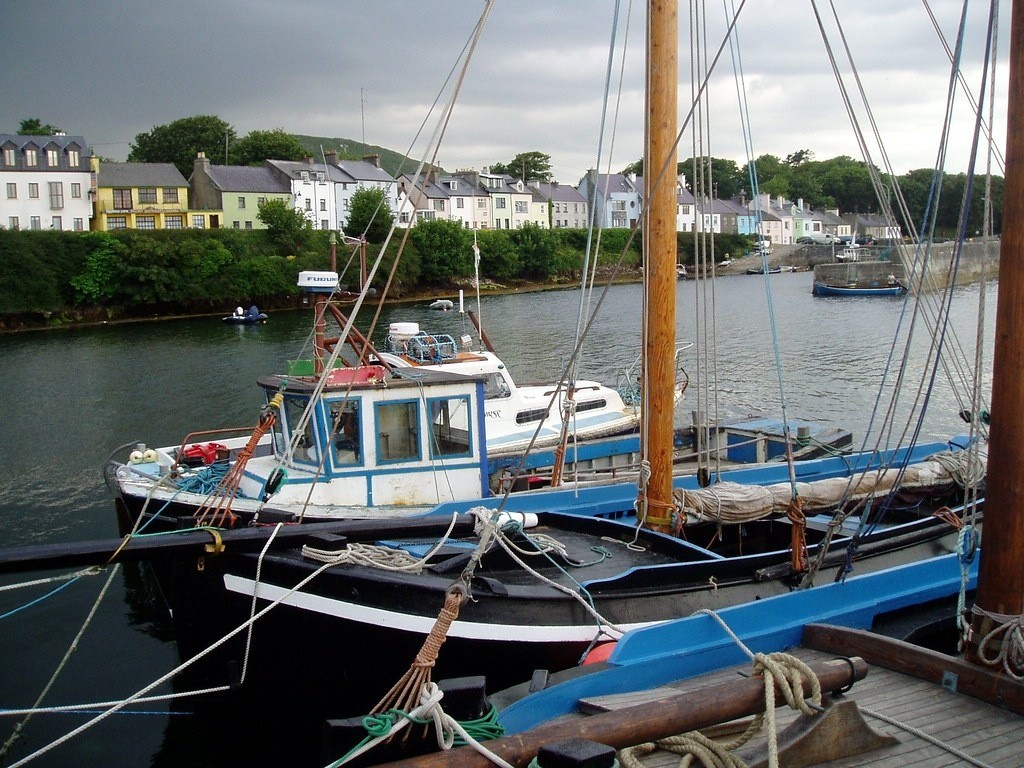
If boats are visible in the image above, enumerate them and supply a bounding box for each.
[746,266,782,275]
[813,272,909,299]
[676,263,688,278]
[102,173,692,568]
[429,300,454,310]
[221,305,268,325]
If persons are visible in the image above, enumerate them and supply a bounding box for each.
[246,302,258,316]
[236,304,243,316]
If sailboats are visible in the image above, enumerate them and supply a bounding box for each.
[183,0,1023,767]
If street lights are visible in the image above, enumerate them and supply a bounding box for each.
[981,198,994,236]
[872,180,892,261]
